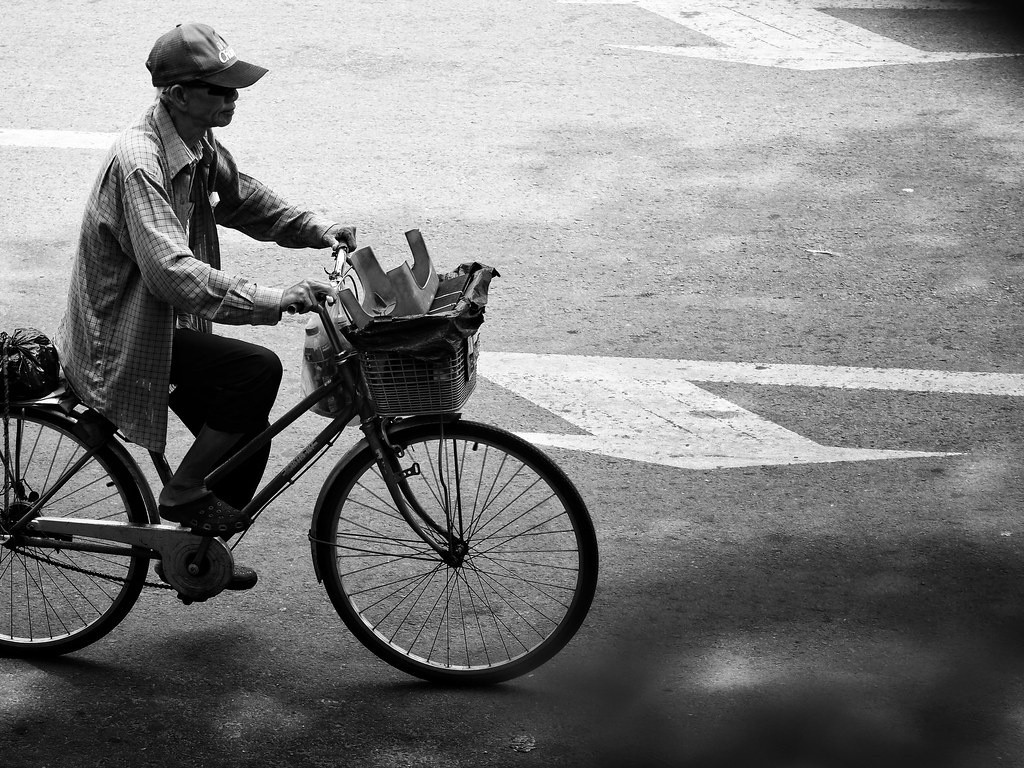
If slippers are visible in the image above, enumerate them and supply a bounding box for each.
[158,490,250,533]
[154,560,258,591]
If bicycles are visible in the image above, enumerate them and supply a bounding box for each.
[1,233,600,689]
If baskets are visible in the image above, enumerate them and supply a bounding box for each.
[357,329,480,415]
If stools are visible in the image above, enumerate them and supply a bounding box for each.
[338,228,440,330]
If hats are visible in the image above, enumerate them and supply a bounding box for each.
[145,23,269,88]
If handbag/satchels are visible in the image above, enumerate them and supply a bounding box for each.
[0,326,60,402]
[300,277,362,427]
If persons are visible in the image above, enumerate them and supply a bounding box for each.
[53,21,354,591]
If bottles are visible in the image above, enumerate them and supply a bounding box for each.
[303,325,346,413]
[334,317,351,351]
